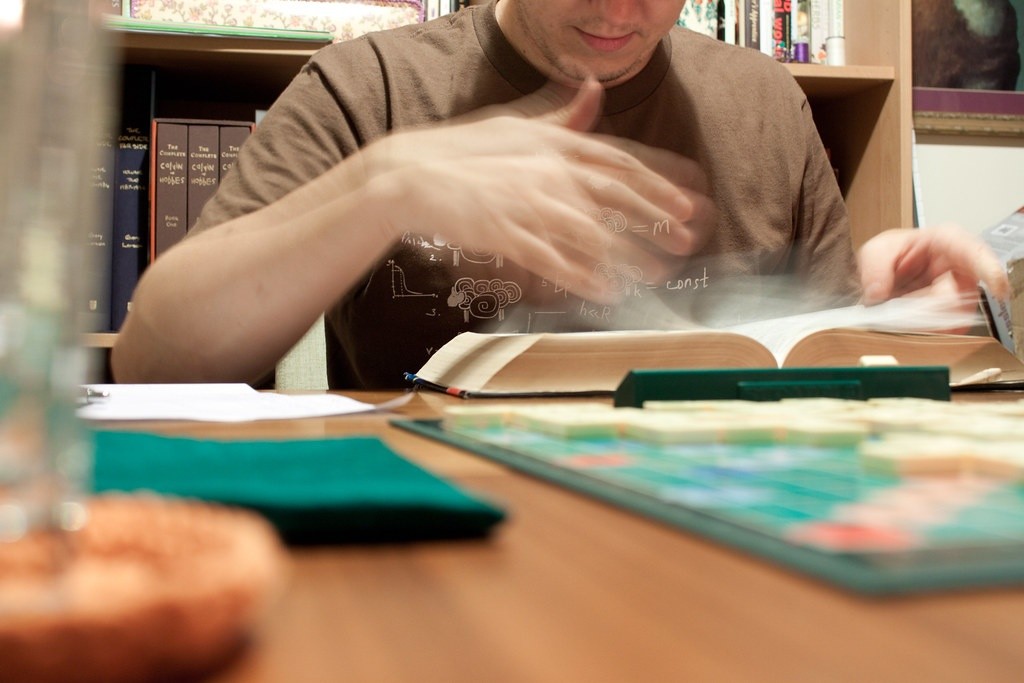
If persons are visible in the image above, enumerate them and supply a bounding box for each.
[112,0,1010,393]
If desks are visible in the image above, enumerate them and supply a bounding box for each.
[80,391,1024,683]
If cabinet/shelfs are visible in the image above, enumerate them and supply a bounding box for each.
[1,0,911,365]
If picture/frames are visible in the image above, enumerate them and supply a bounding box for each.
[912,0,1024,137]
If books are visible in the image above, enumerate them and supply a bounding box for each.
[90,109,257,332]
[680,0,846,66]
[408,291,1024,398]
[422,0,469,23]
